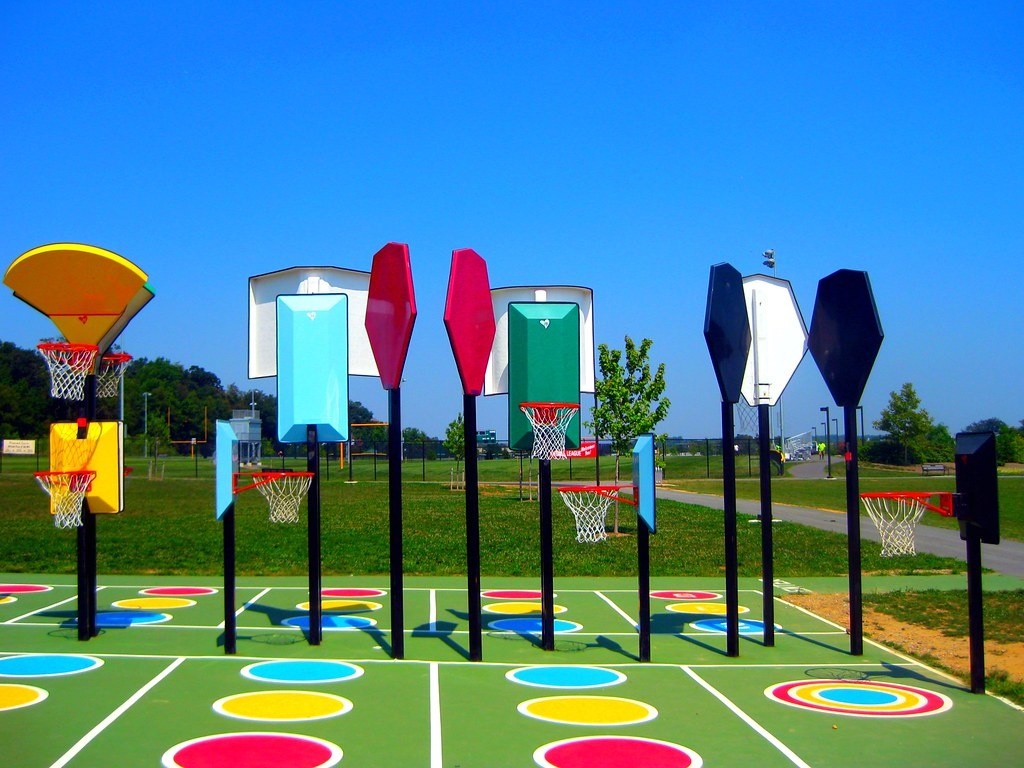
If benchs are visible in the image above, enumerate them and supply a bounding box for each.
[922,464,950,475]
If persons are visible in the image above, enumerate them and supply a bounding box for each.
[734,444,740,457]
[818,440,826,461]
